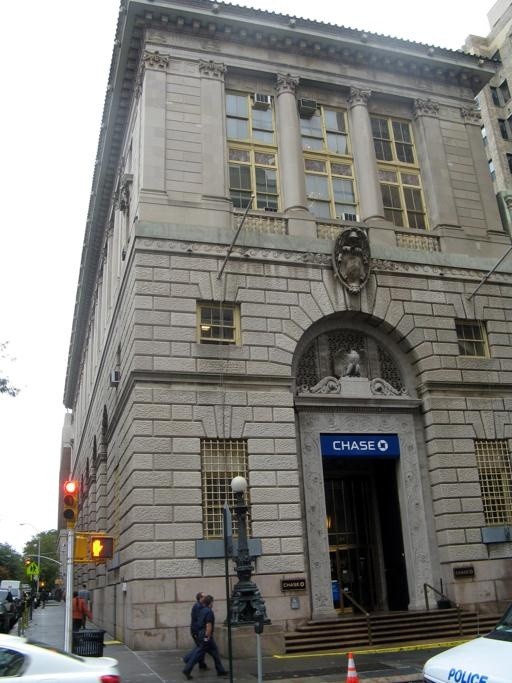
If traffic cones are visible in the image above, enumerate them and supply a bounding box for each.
[343,651,360,683]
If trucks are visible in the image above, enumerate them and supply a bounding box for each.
[419,601,512,681]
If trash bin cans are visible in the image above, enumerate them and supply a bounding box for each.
[72,628,107,657]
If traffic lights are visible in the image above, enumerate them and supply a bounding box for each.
[62,480,83,524]
[90,535,115,562]
[24,557,30,567]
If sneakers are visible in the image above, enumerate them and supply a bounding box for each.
[181,657,206,679]
[217,672,226,676]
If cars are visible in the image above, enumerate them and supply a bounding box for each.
[0,574,53,635]
[0,631,121,683]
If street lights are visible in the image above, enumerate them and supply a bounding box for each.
[216,472,277,628]
[18,522,41,597]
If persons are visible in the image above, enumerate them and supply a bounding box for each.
[15,585,66,609]
[183,591,211,671]
[182,594,230,680]
[76,583,91,630]
[72,590,89,632]
